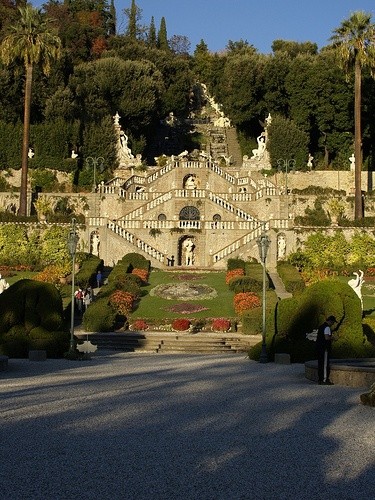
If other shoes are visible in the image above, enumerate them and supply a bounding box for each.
[318,381,334,386]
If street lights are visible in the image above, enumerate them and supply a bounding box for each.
[67,218,81,354]
[86,155,106,193]
[277,157,296,196]
[255,221,272,363]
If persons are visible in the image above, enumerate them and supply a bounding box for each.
[76,285,94,316]
[316,316,338,385]
[96,271,102,288]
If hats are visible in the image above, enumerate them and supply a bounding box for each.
[326,316,339,323]
